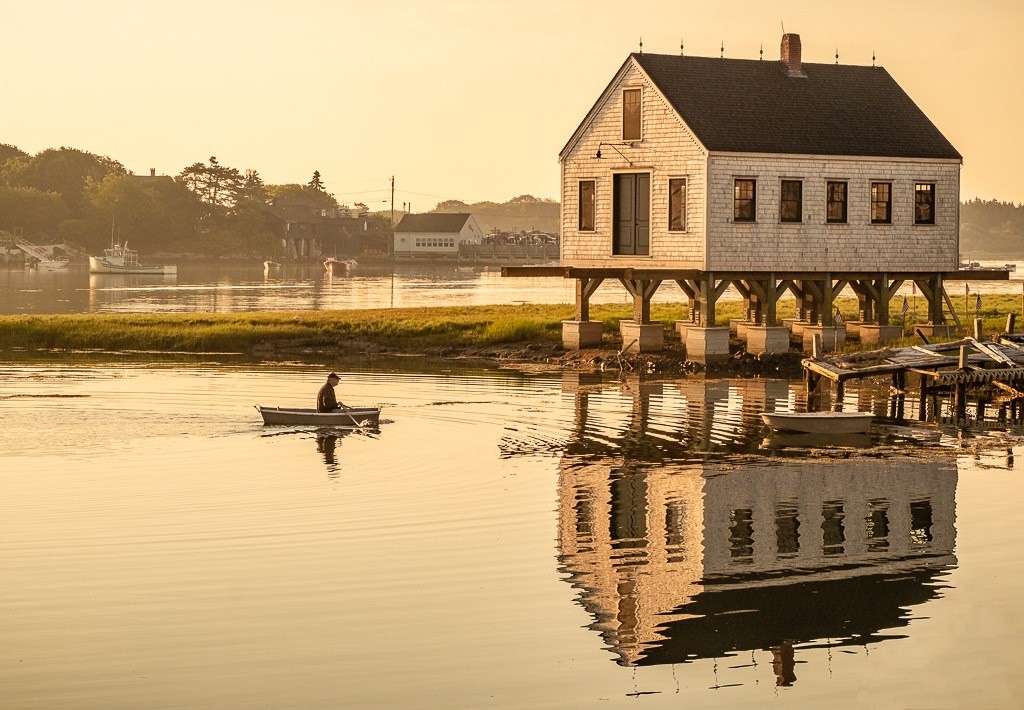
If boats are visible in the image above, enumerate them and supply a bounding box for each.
[87,231,177,275]
[346,260,359,267]
[323,258,350,272]
[263,260,282,269]
[24,253,70,268]
[959,254,1016,272]
[758,431,877,451]
[253,402,380,427]
[759,409,877,434]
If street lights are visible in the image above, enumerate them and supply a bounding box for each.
[111,199,119,249]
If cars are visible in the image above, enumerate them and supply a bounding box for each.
[486,226,558,246]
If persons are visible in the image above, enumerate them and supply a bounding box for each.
[316,373,340,413]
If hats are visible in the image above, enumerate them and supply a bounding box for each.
[328,373,342,380]
